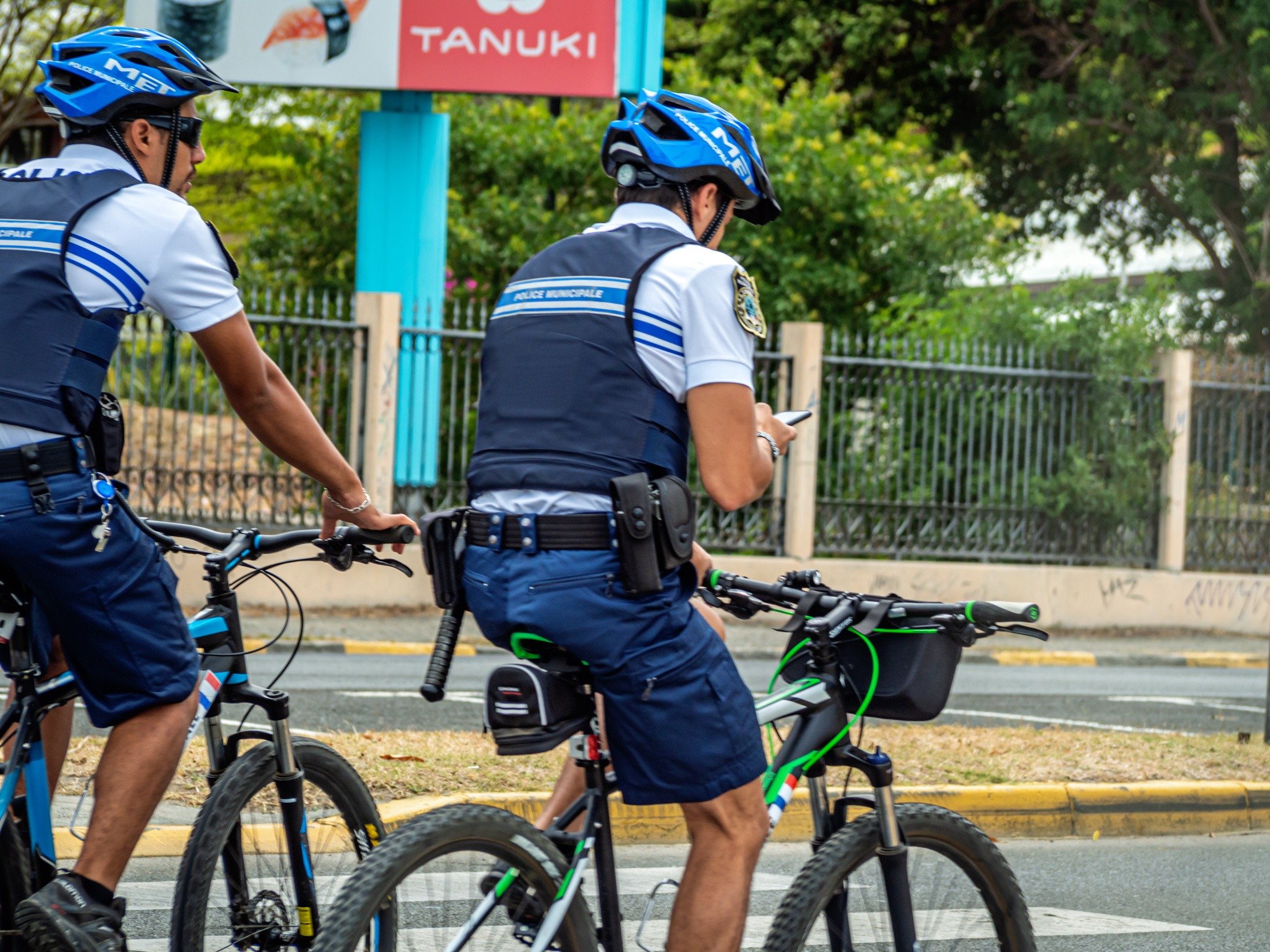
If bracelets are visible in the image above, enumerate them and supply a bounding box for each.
[758,431,780,460]
[322,488,370,512]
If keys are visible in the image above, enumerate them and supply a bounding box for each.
[90,472,114,552]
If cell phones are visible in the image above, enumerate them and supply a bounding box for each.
[773,410,812,426]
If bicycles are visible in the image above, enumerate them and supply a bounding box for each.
[0,509,418,952]
[306,568,1053,952]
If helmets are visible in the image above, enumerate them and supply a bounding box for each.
[600,86,782,225]
[33,26,241,140]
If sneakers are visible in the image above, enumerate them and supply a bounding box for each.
[14,872,127,952]
[479,829,582,931]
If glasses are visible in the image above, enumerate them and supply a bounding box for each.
[116,113,204,150]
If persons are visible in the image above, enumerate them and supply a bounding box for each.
[465,93,799,952]
[0,24,425,952]
